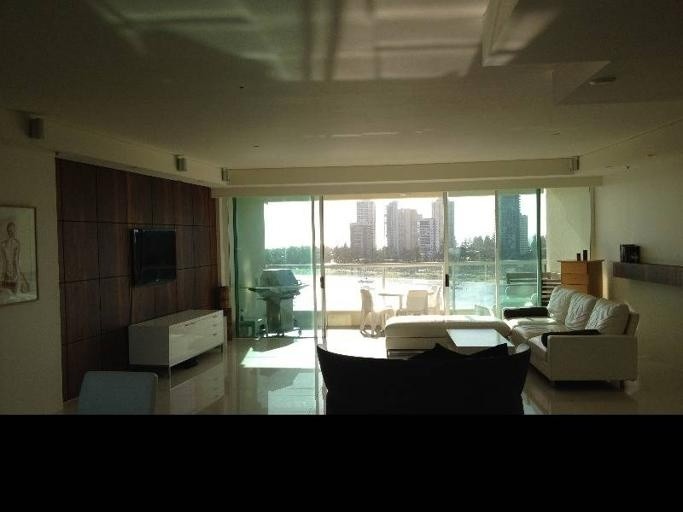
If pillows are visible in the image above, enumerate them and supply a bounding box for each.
[585,298,630,334]
[318,346,532,418]
[547,284,578,324]
[565,292,597,331]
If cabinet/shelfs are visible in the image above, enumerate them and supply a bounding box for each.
[557,256,605,298]
[128,309,227,382]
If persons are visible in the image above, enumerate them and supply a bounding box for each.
[0,221,22,290]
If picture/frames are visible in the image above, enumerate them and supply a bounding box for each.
[0,204,41,307]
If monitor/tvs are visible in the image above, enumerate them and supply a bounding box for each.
[131,227,176,288]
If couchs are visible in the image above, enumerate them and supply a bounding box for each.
[504,284,641,390]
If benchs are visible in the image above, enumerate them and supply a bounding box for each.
[384,315,508,357]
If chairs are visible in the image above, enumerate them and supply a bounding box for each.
[360,284,441,336]
